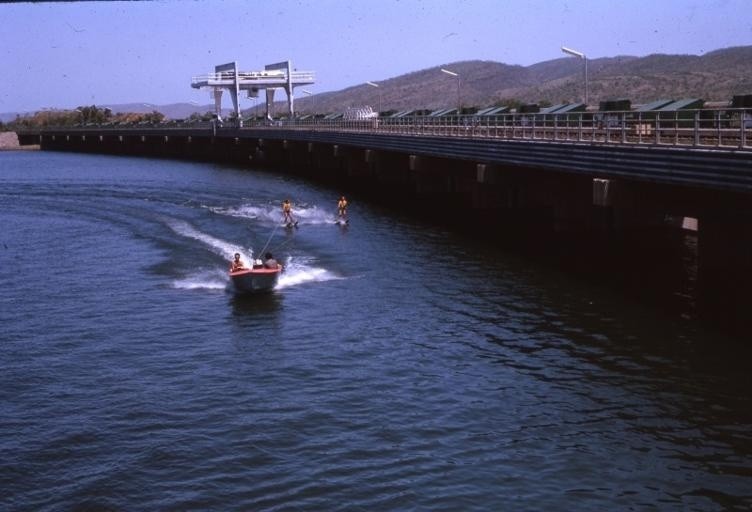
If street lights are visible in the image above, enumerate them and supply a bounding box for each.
[441,69,461,114]
[561,47,587,104]
[366,81,381,112]
[248,97,257,121]
[303,90,314,121]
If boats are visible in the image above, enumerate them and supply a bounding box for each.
[228,259,283,290]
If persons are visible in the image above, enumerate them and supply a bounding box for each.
[261,252,277,269]
[231,253,244,273]
[337,195,347,220]
[282,199,295,224]
